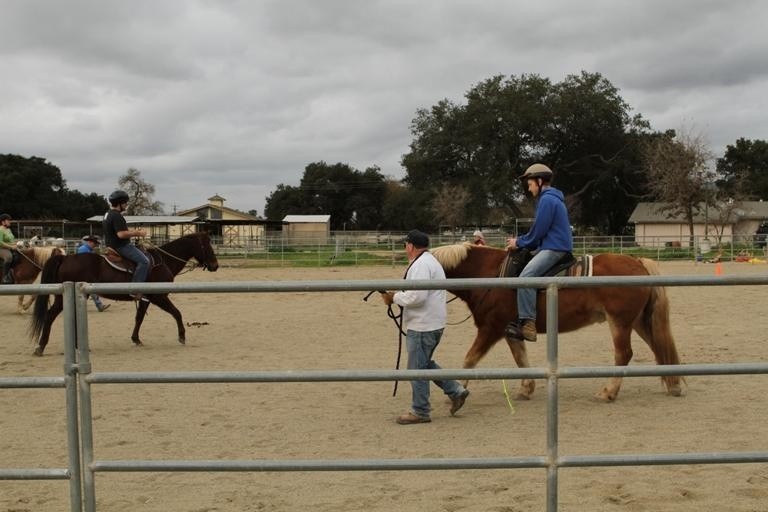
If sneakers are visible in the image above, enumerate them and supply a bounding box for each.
[504,317,538,342]
[98,302,113,313]
[396,412,432,425]
[449,390,471,416]
[128,292,150,303]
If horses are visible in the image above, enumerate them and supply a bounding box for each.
[32,232,218,357]
[404,243,687,402]
[0,241,64,315]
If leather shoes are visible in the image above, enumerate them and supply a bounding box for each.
[2,274,12,285]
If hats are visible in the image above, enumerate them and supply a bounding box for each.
[82,233,101,248]
[472,229,484,239]
[0,212,11,222]
[108,189,131,205]
[518,163,554,181]
[402,240,408,248]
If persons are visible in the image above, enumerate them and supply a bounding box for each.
[78,235,111,313]
[382,231,470,425]
[507,163,573,343]
[0,213,18,283]
[103,191,151,299]
[473,230,485,246]
